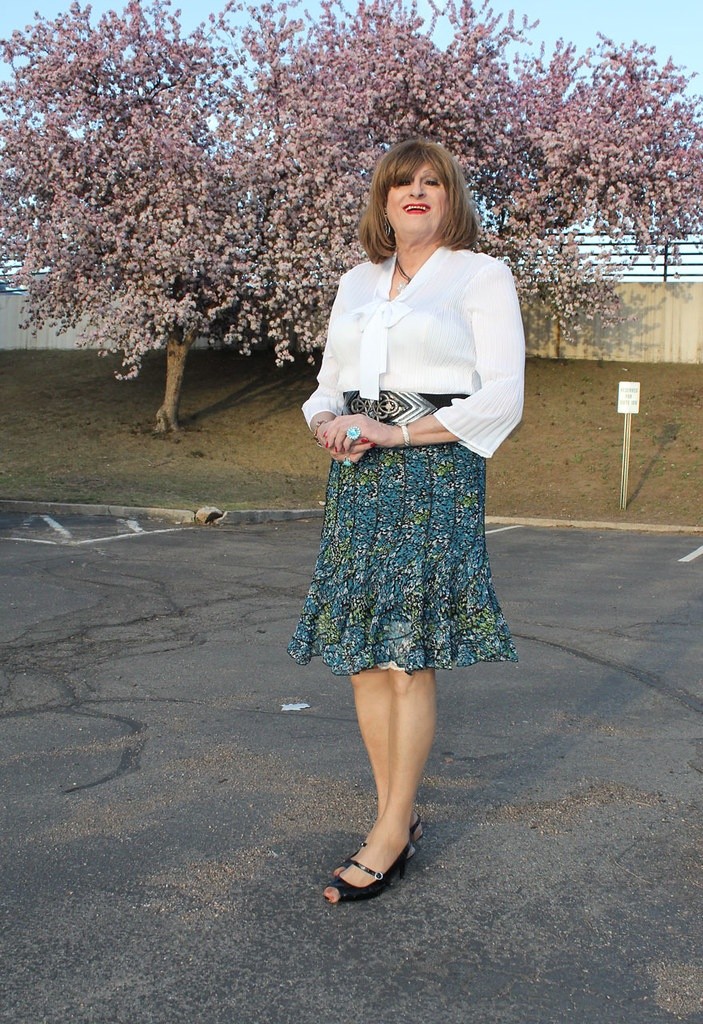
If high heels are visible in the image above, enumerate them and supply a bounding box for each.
[321,842,415,904]
[332,810,423,880]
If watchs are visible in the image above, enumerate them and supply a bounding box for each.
[396,421,410,448]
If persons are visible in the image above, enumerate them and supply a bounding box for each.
[285,139,526,907]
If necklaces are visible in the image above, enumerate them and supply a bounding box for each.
[395,258,412,293]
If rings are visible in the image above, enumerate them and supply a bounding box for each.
[346,427,361,440]
[342,458,353,467]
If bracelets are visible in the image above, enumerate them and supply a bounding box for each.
[312,420,327,448]
